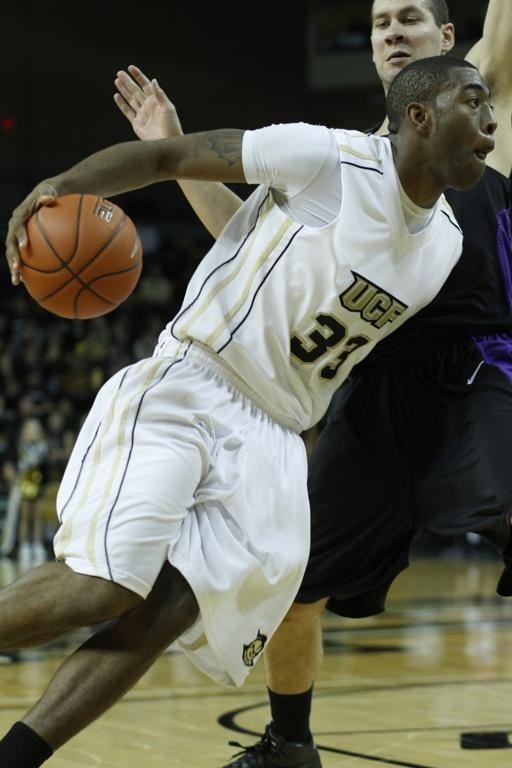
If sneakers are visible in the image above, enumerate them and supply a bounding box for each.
[221,723,322,767]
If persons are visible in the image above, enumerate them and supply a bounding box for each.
[0,53,497,767]
[112,1,512,767]
[0,224,185,567]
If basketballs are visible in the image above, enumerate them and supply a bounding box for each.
[19,194,142,321]
